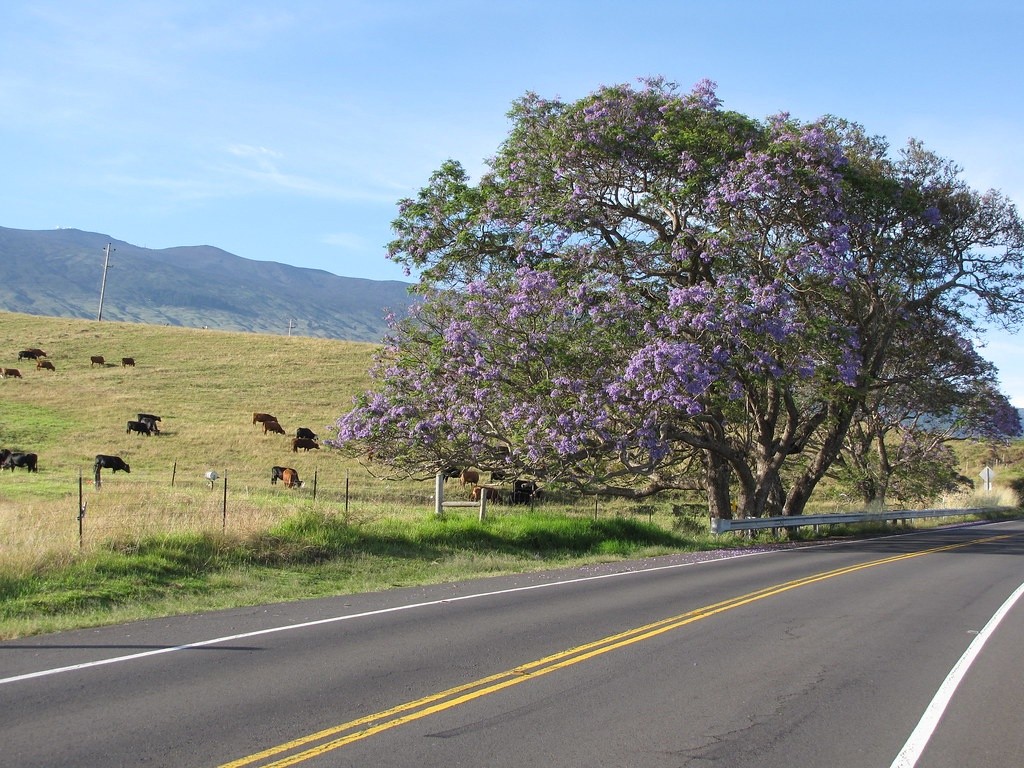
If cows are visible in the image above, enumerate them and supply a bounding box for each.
[93,454,131,474]
[271,466,303,490]
[126,413,162,437]
[252,412,321,453]
[90,356,106,365]
[458,470,532,505]
[122,358,136,367]
[0,348,56,379]
[0,448,38,473]
